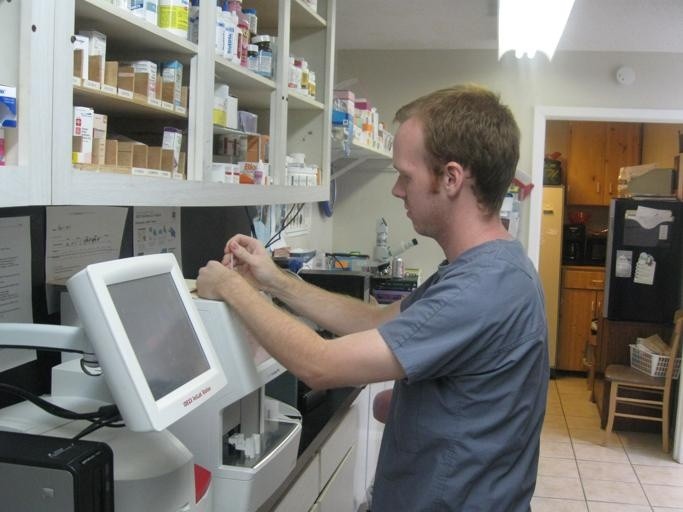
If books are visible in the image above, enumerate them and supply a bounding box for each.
[632,196,676,201]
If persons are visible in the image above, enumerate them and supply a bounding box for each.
[196,85,549,512]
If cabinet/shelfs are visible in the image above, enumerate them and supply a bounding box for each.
[566,120,642,207]
[0,0,335,207]
[595,318,673,434]
[557,270,605,373]
[270,372,395,512]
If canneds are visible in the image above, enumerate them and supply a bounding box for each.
[392,256,404,278]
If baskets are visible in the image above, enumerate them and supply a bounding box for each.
[630,345,681,380]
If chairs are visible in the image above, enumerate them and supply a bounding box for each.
[602,308,683,453]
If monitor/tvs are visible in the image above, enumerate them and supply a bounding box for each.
[68,253,227,430]
[297,269,370,308]
